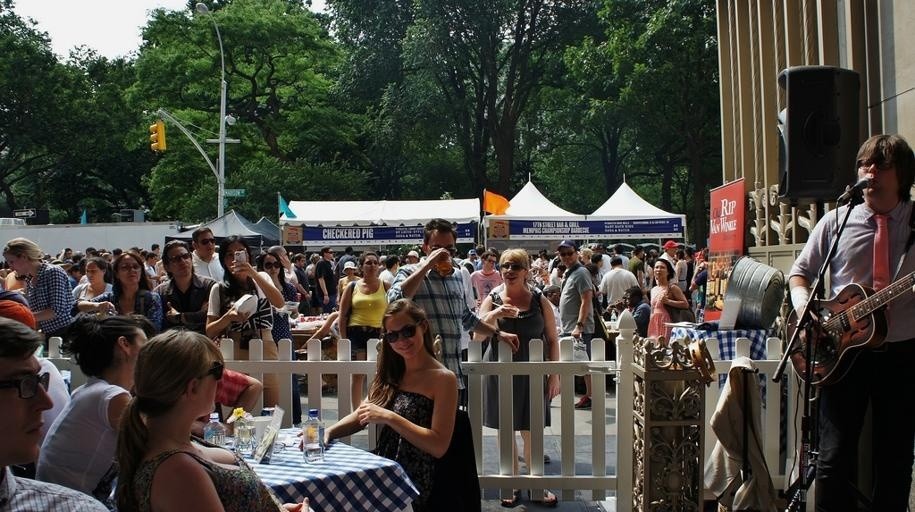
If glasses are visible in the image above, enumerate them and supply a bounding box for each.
[365,261,380,265]
[387,321,422,344]
[264,261,281,269]
[501,263,523,271]
[560,252,573,257]
[327,251,335,254]
[170,252,192,262]
[859,160,892,171]
[431,246,457,255]
[120,263,143,272]
[201,238,217,245]
[1,373,51,398]
[200,358,226,382]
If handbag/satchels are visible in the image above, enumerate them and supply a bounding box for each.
[594,309,610,340]
[662,286,696,324]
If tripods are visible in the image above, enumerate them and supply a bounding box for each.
[783,202,874,512]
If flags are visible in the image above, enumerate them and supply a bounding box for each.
[483,190,511,215]
[279,195,296,218]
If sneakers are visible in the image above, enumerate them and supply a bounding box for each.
[575,396,592,410]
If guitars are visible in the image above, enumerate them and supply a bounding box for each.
[785,269,915,386]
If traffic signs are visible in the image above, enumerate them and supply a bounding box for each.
[12,209,37,219]
[224,187,247,198]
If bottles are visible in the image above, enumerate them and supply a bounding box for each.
[203,411,226,447]
[302,408,324,461]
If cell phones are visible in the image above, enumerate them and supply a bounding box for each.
[234,251,246,274]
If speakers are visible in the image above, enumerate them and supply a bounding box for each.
[776,66,861,203]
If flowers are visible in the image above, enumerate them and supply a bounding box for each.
[233,407,246,423]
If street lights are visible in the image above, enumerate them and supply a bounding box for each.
[189,2,227,223]
[222,114,237,127]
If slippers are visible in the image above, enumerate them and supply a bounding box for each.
[528,488,558,505]
[502,488,522,507]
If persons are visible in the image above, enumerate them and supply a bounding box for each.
[787,134,915,512]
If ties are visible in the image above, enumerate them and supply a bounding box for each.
[871,212,891,289]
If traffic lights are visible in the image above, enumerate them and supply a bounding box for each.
[148,120,166,154]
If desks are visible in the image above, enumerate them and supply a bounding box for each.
[662,324,788,412]
[220,427,424,512]
[289,312,336,356]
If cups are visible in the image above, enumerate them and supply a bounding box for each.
[230,409,281,464]
[437,256,452,273]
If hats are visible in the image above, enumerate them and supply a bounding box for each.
[663,240,679,250]
[557,239,576,251]
[342,261,359,275]
[468,249,476,255]
[405,250,419,259]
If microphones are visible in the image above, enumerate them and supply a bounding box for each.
[836,175,873,202]
